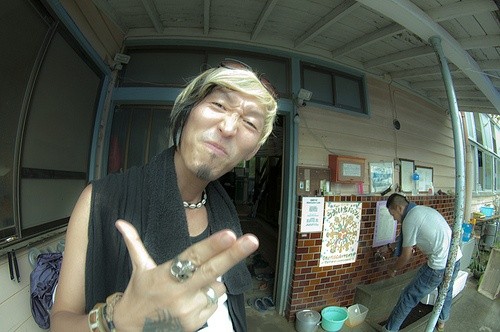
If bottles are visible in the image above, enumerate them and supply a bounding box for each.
[412,174,420,196]
[427,182,434,196]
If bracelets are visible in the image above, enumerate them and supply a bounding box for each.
[88,293,124,332]
[392,267,397,272]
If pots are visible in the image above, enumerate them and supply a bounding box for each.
[294,310,323,332]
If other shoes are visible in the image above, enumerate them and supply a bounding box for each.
[249,254,274,291]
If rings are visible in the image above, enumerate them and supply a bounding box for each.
[170,259,196,284]
[202,287,219,308]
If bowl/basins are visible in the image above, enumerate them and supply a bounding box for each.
[344,304,369,328]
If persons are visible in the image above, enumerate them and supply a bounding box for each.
[377,193,463,332]
[49,59,277,332]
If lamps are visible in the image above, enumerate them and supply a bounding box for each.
[112,53,131,69]
[297,89,312,105]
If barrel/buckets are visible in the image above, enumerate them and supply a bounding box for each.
[480,207,493,217]
[461,223,473,241]
[321,305,349,332]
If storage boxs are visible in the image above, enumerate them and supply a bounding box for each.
[345,304,369,328]
[420,270,468,305]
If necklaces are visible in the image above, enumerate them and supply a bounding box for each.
[181,190,207,209]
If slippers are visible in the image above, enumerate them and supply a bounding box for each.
[247,298,269,313]
[262,296,275,310]
[435,324,445,332]
